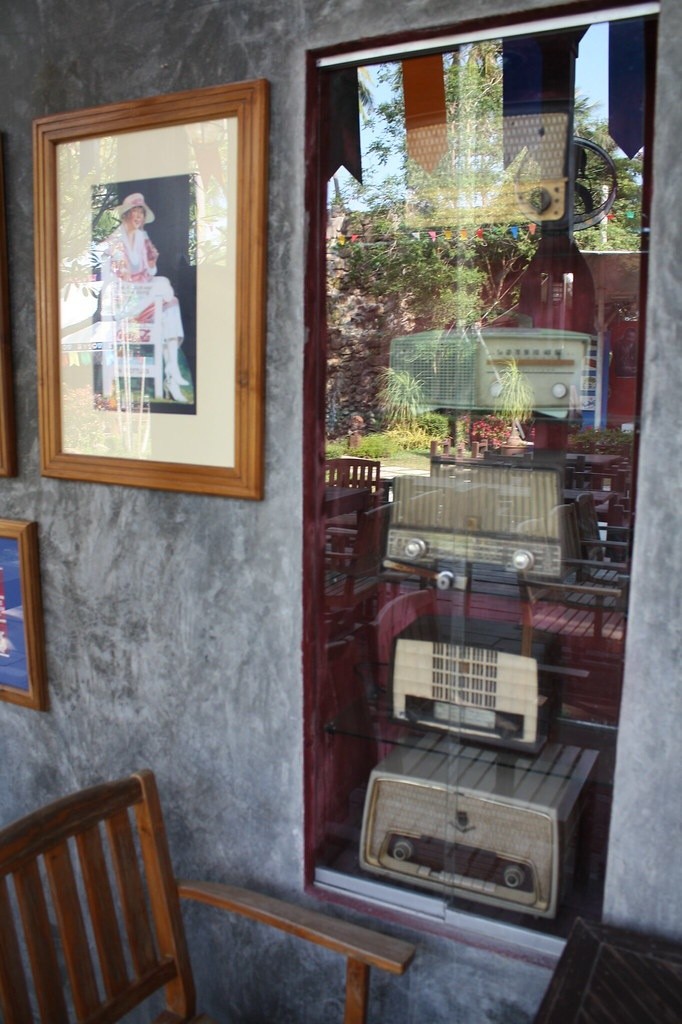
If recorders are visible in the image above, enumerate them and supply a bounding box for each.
[391,613,563,754]
[379,98,604,594]
[358,726,601,919]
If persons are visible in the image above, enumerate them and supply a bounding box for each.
[93,193,190,402]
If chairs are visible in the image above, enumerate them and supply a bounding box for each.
[0,770,416,1024]
[324,404,635,630]
[100,255,162,402]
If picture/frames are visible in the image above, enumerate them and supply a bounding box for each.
[30,79,273,502]
[0,517,51,713]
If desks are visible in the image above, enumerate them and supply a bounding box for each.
[324,484,369,524]
[531,915,682,1024]
[518,450,623,491]
